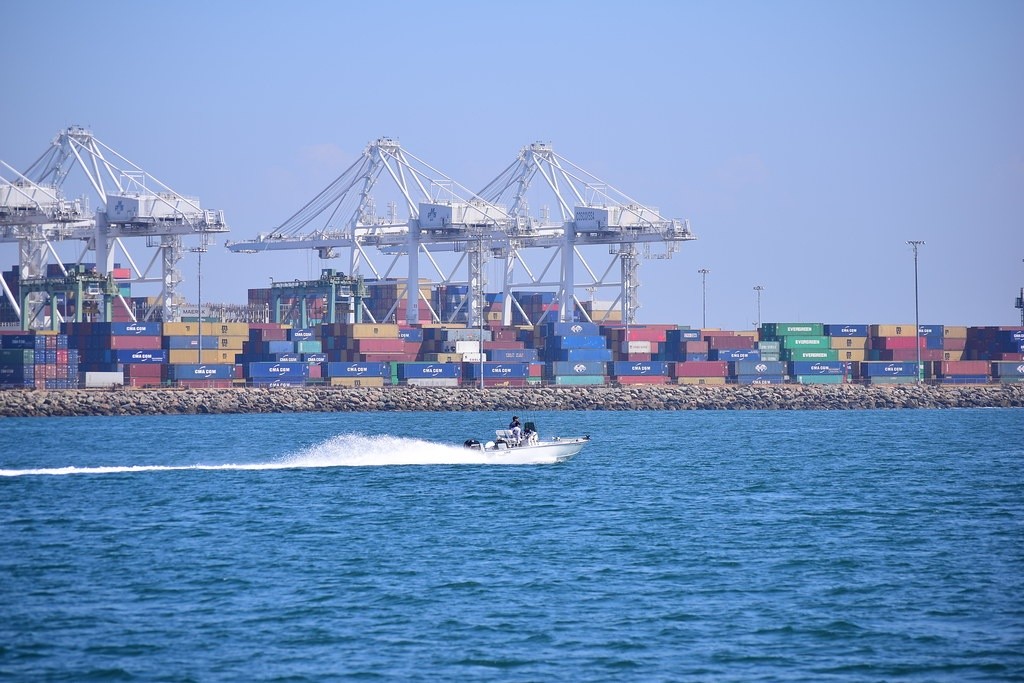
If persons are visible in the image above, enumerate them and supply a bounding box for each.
[510,416,519,429]
[512,422,521,444]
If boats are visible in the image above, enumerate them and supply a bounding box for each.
[465,430,590,461]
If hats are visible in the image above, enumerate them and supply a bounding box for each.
[513,416,519,420]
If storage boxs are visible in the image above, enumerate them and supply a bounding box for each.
[1,262,1024,389]
